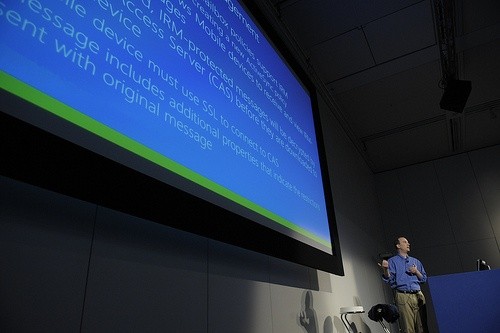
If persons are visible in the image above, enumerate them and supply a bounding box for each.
[377,235,428,333]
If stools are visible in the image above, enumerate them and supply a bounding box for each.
[339,304,400,333]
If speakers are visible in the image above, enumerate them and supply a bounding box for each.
[439,80,472,113]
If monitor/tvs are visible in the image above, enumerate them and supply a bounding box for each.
[476,259,491,271]
